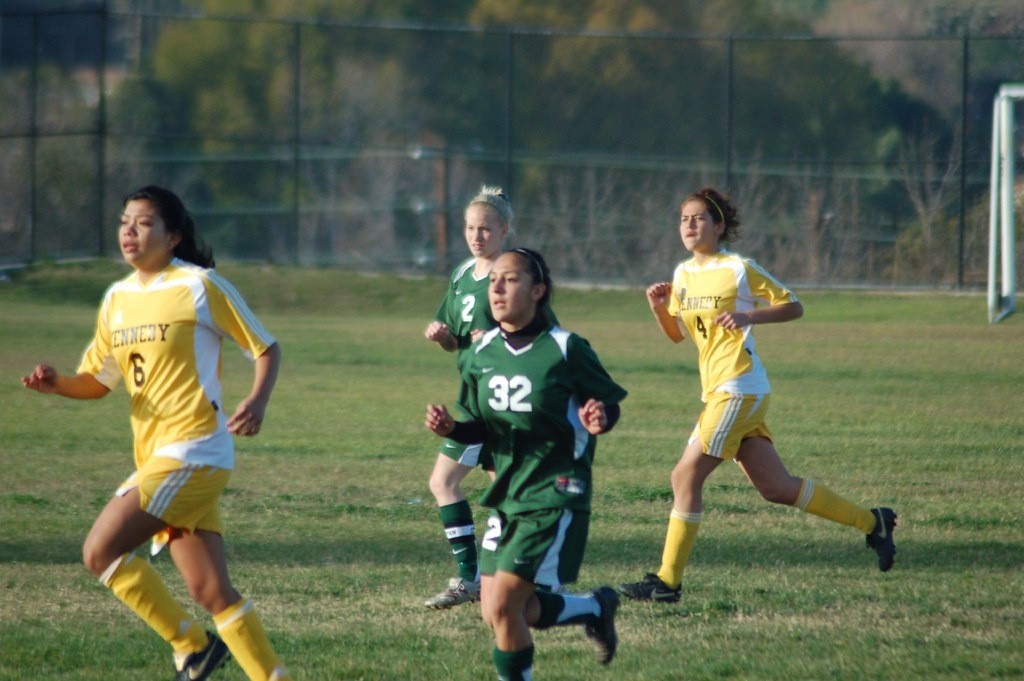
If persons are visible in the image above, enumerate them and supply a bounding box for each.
[618,188,898,603]
[20,183,292,680]
[423,183,565,611]
[426,248,628,680]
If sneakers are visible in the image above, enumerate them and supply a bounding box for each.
[424,577,482,610]
[585,586,620,664]
[866,506,897,571]
[618,572,682,603]
[173,630,230,681]
